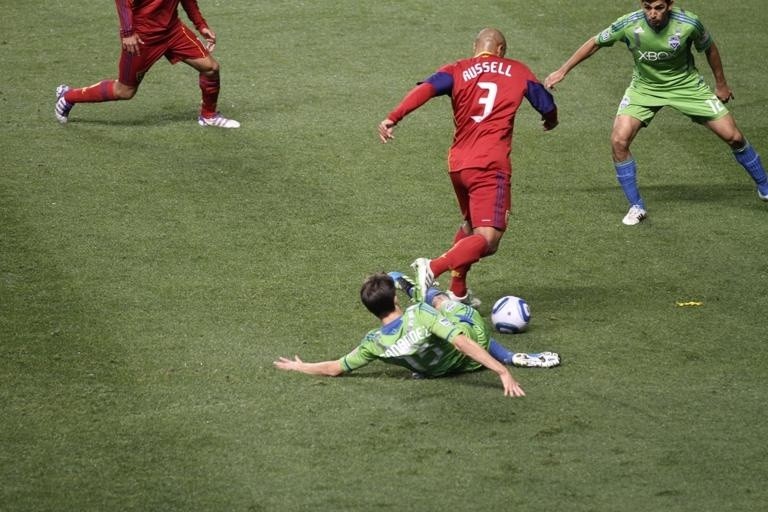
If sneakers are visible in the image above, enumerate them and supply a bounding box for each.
[445,289,482,308]
[54,84,74,124]
[622,205,648,226]
[757,188,768,201]
[198,110,240,128]
[387,271,416,300]
[512,351,561,368]
[410,257,435,304]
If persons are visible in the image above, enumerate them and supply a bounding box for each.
[53,0,242,130]
[539,3,768,223]
[271,269,562,403]
[375,28,560,306]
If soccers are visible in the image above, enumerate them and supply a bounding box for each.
[489,295,531,336]
